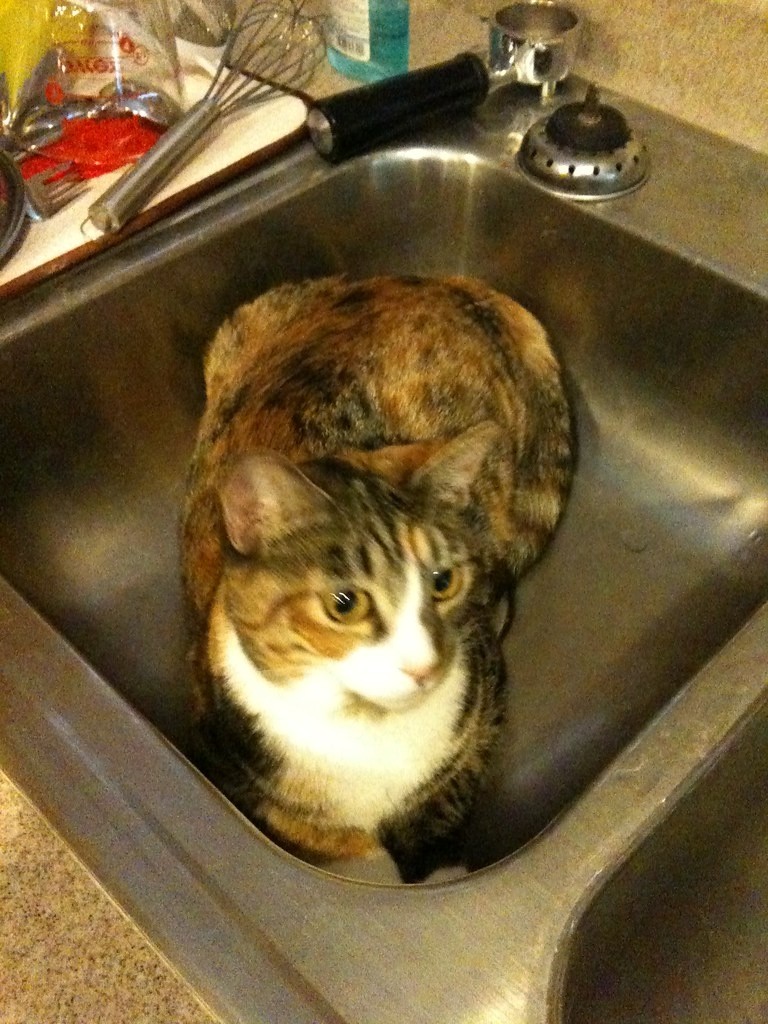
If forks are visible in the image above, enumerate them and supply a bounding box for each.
[23,161,92,222]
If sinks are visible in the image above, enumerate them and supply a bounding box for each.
[464,698,768,1023]
[0,149,768,908]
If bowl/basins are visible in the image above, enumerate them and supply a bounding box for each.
[0,148,27,261]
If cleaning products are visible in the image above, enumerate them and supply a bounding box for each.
[324,0,410,84]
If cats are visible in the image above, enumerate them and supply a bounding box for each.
[179,273,579,888]
